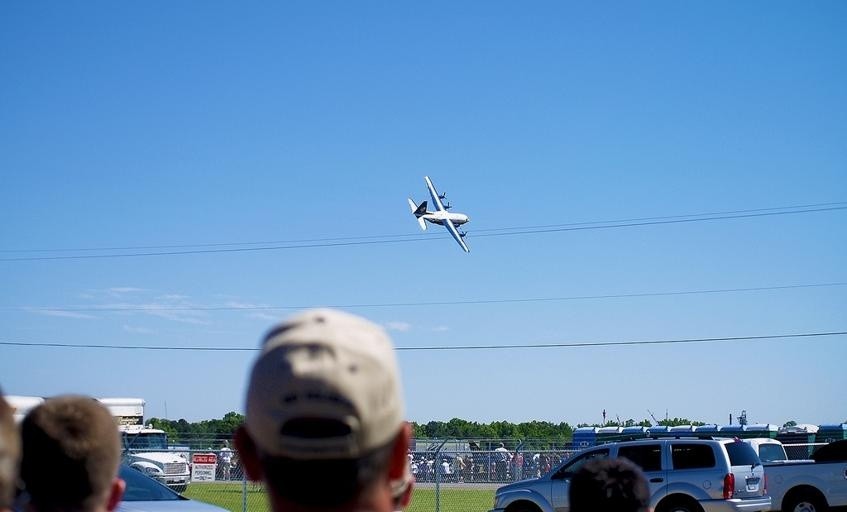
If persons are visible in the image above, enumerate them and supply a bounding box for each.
[16,394,126,512]
[207,440,244,481]
[408,442,570,483]
[231,308,411,512]
[568,456,651,512]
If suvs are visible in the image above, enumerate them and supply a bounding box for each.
[486,436,776,512]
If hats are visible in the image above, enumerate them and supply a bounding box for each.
[244,307,404,460]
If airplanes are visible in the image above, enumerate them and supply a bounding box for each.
[408,174,473,254]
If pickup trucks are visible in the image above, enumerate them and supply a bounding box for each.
[712,438,846,512]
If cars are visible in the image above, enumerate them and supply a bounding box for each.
[113,463,231,511]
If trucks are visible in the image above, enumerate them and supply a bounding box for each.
[5,396,191,492]
